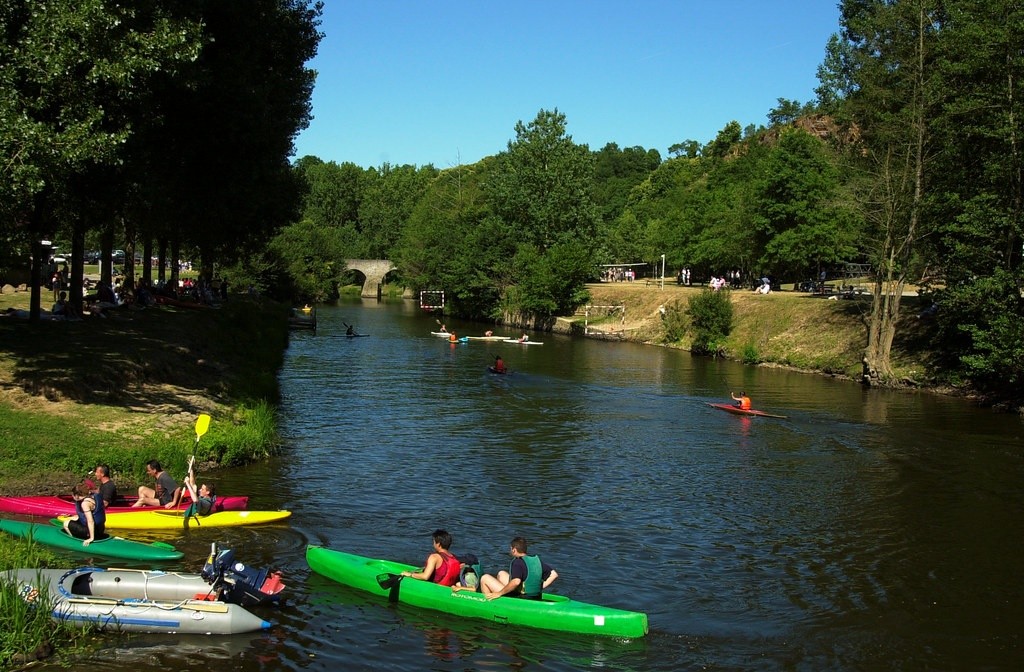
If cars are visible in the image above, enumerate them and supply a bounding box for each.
[133,252,142,265]
[52,253,67,264]
[110,249,124,264]
[83,250,102,265]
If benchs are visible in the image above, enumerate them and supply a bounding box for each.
[812,284,835,294]
[678,276,711,286]
[844,270,872,278]
[646,280,665,288]
[709,282,730,292]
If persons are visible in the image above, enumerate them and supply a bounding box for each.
[709,270,740,292]
[523,333,529,342]
[843,286,855,299]
[248,283,258,301]
[346,325,353,335]
[485,329,492,336]
[682,268,690,284]
[480,536,558,600]
[606,268,635,282]
[731,391,751,409]
[132,459,181,510]
[494,355,504,369]
[910,297,937,320]
[401,529,460,586]
[440,324,446,332]
[660,305,665,320]
[89,465,117,507]
[451,554,483,592]
[450,331,457,341]
[184,458,216,517]
[800,270,826,292]
[64,483,106,547]
[753,276,782,294]
[6,258,230,321]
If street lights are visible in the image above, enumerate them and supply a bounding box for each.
[661,254,666,293]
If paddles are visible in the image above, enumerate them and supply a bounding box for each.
[375,553,479,590]
[176,413,211,515]
[67,597,230,613]
[343,322,357,334]
[84,479,96,490]
[48,517,177,550]
[436,318,443,328]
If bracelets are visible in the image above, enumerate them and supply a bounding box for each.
[410,573,413,577]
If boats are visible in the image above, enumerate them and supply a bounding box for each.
[488,365,507,373]
[306,543,648,638]
[429,331,545,345]
[332,333,370,337]
[702,400,787,419]
[0,543,286,635]
[0,495,249,518]
[0,519,186,561]
[55,509,293,529]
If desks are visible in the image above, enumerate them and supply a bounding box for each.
[847,263,871,277]
